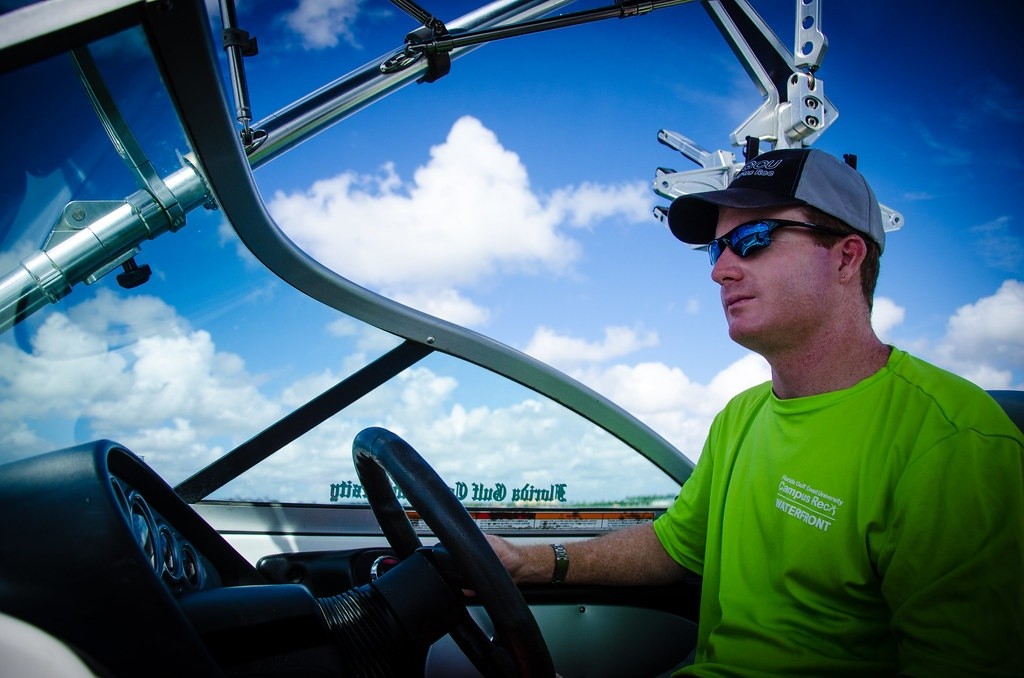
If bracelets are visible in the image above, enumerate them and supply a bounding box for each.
[549,543,570,585]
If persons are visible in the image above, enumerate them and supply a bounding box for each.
[461,149,1024,678]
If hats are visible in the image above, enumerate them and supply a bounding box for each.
[667,149,885,256]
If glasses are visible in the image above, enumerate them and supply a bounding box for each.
[707,217,875,266]
